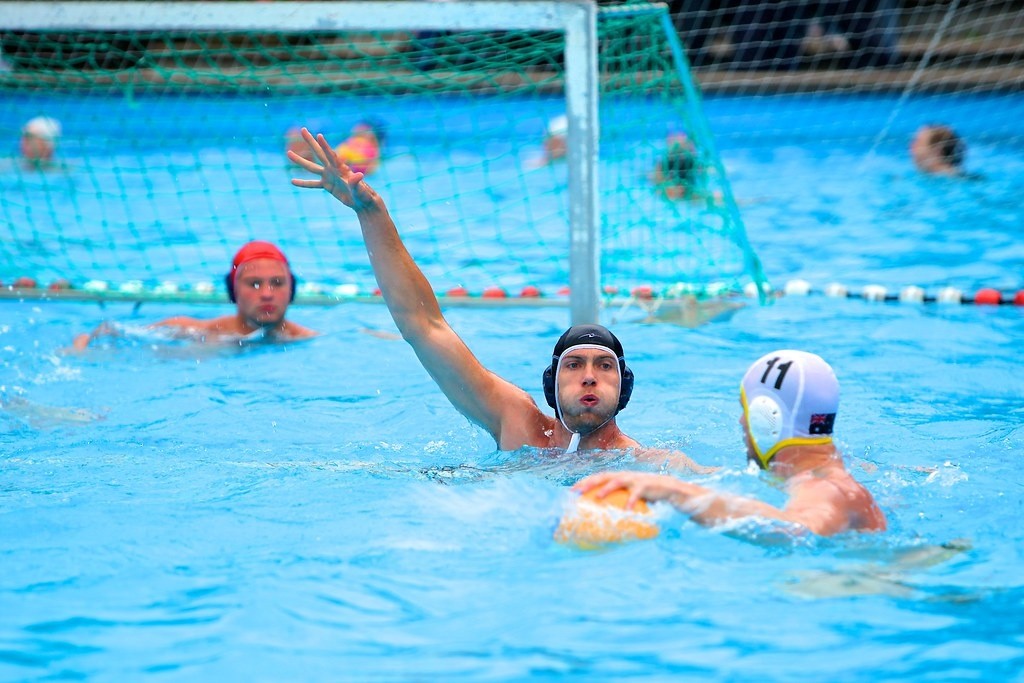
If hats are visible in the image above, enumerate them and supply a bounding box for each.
[552,324,625,434]
[28,115,60,143]
[231,240,288,286]
[741,348,840,463]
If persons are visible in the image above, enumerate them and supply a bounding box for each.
[908,121,965,180]
[20,116,61,168]
[70,241,318,352]
[523,113,567,167]
[649,127,706,203]
[286,127,942,481]
[570,351,889,535]
[275,117,386,175]
